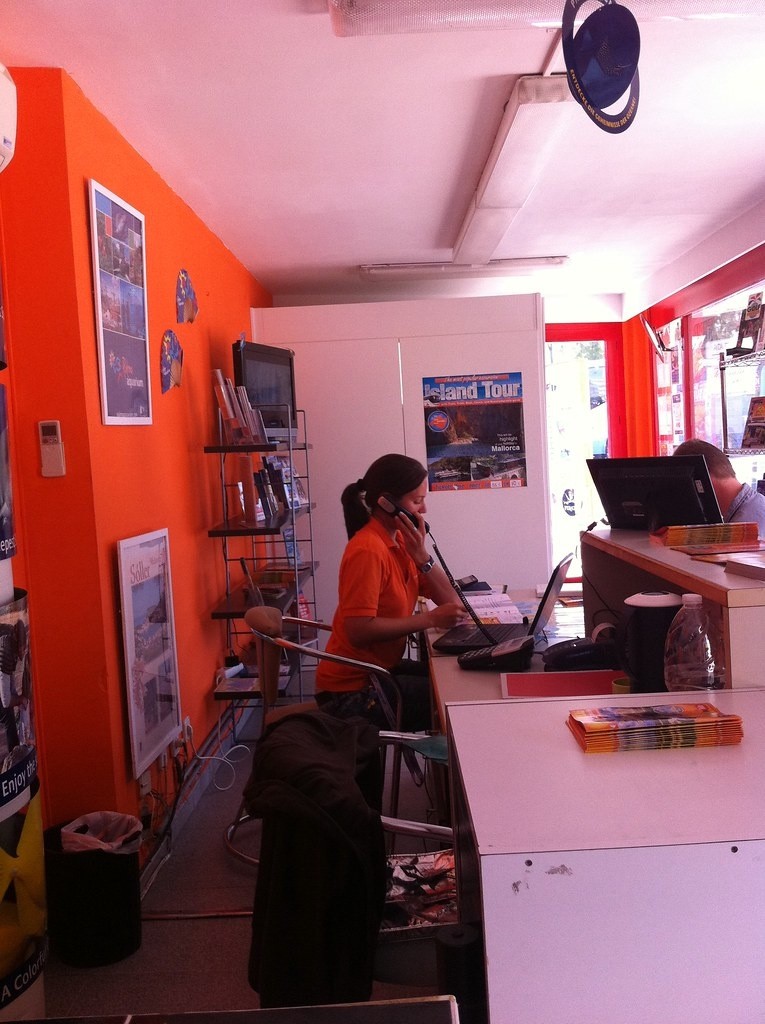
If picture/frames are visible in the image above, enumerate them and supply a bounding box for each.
[116,526,181,779]
[88,179,154,426]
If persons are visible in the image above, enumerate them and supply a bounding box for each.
[674,438,765,541]
[316,454,469,733]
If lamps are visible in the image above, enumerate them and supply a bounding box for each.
[453,56,571,263]
[359,257,570,282]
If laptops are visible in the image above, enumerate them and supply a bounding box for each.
[433,552,574,655]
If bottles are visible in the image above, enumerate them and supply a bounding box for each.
[664,594,726,691]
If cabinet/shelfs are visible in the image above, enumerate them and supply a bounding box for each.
[721,350,765,456]
[204,404,319,742]
[251,293,546,665]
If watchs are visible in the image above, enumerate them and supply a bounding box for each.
[419,556,435,573]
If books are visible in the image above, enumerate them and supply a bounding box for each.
[290,590,313,627]
[284,524,303,565]
[212,368,308,521]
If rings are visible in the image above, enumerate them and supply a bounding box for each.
[412,527,417,532]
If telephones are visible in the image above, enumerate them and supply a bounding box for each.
[377,495,430,536]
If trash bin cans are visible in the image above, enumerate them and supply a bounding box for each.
[43,810,146,969]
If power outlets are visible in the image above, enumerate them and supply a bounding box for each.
[170,736,179,757]
[184,716,193,742]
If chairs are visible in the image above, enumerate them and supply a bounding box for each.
[225,604,454,959]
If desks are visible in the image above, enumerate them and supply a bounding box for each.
[422,588,765,1024]
[581,530,765,688]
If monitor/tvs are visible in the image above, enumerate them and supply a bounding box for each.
[234,339,299,427]
[587,455,725,530]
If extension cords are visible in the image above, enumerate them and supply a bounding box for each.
[217,662,244,680]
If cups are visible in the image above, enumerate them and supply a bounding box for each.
[611,676,631,694]
